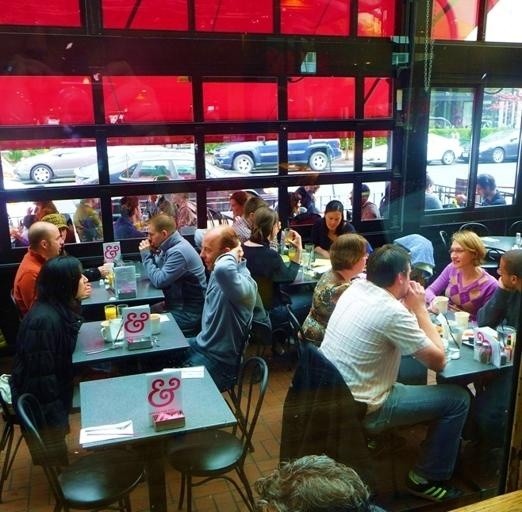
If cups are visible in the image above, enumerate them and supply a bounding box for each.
[104,304,116,321]
[150,313,161,335]
[118,304,127,320]
[454,310,471,328]
[435,296,449,316]
[302,253,312,280]
[284,228,293,248]
[305,243,315,263]
[288,248,297,262]
[104,263,115,273]
[101,321,111,343]
[449,326,464,361]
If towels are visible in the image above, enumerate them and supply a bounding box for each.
[163,365,205,379]
[159,314,171,322]
[79,420,133,444]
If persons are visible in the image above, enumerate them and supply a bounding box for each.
[146,194,176,222]
[305,200,355,257]
[73,198,102,240]
[22,200,60,225]
[457,174,506,207]
[318,243,473,503]
[171,192,197,228]
[139,216,208,330]
[453,249,522,490]
[425,230,495,325]
[164,225,259,393]
[253,455,383,512]
[230,191,249,219]
[120,195,147,231]
[296,185,323,216]
[115,203,148,239]
[426,175,444,209]
[295,233,369,348]
[276,192,302,226]
[14,221,92,316]
[40,213,68,258]
[18,215,37,247]
[11,254,88,436]
[240,207,318,358]
[349,184,380,219]
[232,196,281,252]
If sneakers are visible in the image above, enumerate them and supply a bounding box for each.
[406,469,465,502]
[368,438,407,457]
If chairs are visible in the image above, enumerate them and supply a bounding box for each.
[172,357,269,512]
[17,394,146,512]
[508,219,522,236]
[172,323,257,454]
[460,223,489,236]
[439,230,452,263]
[482,247,507,279]
[306,343,401,504]
[0,375,63,503]
[10,285,23,323]
[284,304,318,362]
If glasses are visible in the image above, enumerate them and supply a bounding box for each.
[448,248,471,255]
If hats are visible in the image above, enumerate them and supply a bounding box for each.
[41,214,69,229]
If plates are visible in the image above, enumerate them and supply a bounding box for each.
[465,330,474,351]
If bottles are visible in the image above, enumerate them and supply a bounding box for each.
[481,341,491,364]
[504,334,512,362]
[474,340,484,361]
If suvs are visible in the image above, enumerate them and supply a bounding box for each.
[212,139,345,172]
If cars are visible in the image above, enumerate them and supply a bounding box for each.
[80,157,279,222]
[363,133,463,167]
[12,146,130,184]
[455,129,519,163]
